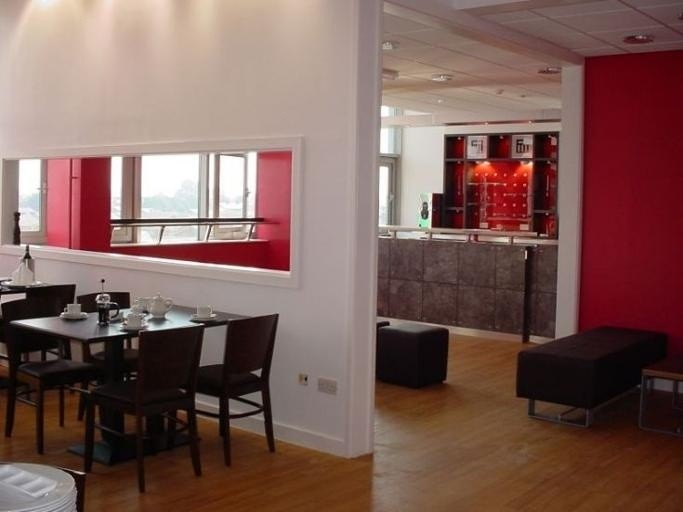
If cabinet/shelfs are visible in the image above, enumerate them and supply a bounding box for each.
[442,131,558,233]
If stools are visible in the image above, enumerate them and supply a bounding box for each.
[380,322,448,388]
[637,358,682,437]
[376,319,389,378]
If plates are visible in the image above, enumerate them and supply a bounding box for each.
[1,280,42,286]
[119,323,151,330]
[0,465,58,497]
[189,313,221,321]
[58,311,88,320]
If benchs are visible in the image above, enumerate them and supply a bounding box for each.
[516,326,670,428]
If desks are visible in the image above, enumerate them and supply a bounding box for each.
[10,305,252,466]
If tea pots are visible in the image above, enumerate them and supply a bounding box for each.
[146,292,173,319]
[10,262,33,285]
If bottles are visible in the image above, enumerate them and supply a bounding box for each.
[12,212,20,245]
[21,245,31,268]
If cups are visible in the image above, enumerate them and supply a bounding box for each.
[131,304,144,314]
[126,313,144,328]
[196,303,213,318]
[94,294,120,327]
[62,303,81,316]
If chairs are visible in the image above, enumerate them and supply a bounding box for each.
[166,313,280,466]
[54,464,87,512]
[84,323,204,493]
[75,292,140,374]
[2,295,104,455]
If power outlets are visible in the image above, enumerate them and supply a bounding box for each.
[327,380,338,394]
